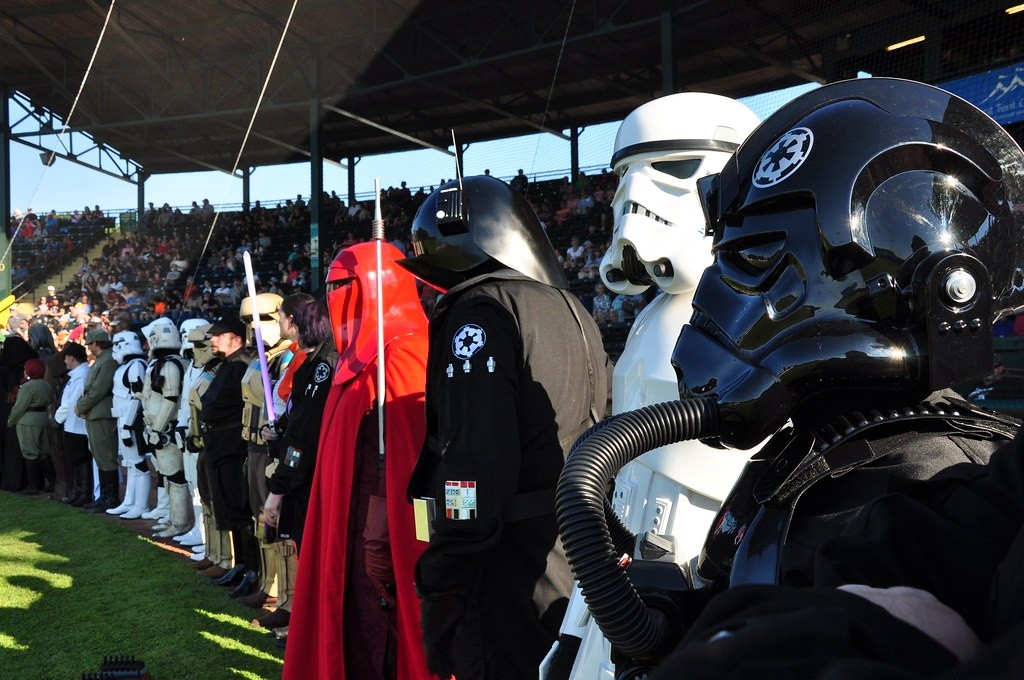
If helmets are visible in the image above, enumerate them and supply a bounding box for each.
[141,317,182,358]
[599,91,763,296]
[670,74,1023,448]
[324,239,430,386]
[180,318,210,357]
[112,328,146,364]
[188,316,219,368]
[394,174,571,291]
[239,293,284,348]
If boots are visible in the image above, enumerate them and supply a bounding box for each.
[12,460,206,561]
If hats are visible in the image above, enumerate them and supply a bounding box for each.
[206,316,246,341]
[85,330,109,345]
[62,342,86,355]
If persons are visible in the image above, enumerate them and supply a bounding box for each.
[0,320,121,514]
[194,318,261,598]
[392,178,613,680]
[510,170,664,323]
[283,240,455,680]
[0,168,493,363]
[237,293,300,625]
[102,316,235,579]
[557,76,1024,680]
[536,91,792,680]
[248,292,340,647]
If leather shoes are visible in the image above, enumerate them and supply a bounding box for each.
[251,607,291,627]
[273,627,289,639]
[237,590,277,607]
[190,557,231,576]
[206,567,259,598]
